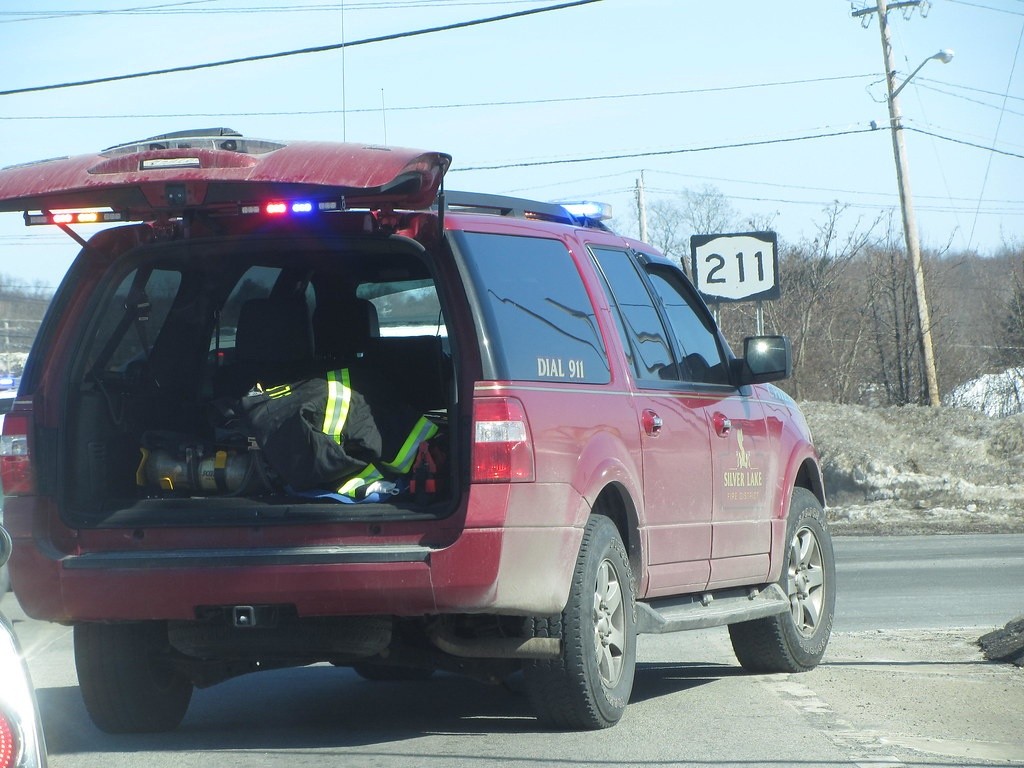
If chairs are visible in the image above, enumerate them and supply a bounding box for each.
[232,296,313,382]
[309,289,378,358]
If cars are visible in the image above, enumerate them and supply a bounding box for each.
[0,526,50,768]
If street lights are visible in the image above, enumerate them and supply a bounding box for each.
[889,49,954,405]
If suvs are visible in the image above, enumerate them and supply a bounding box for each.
[0,126,835,728]
